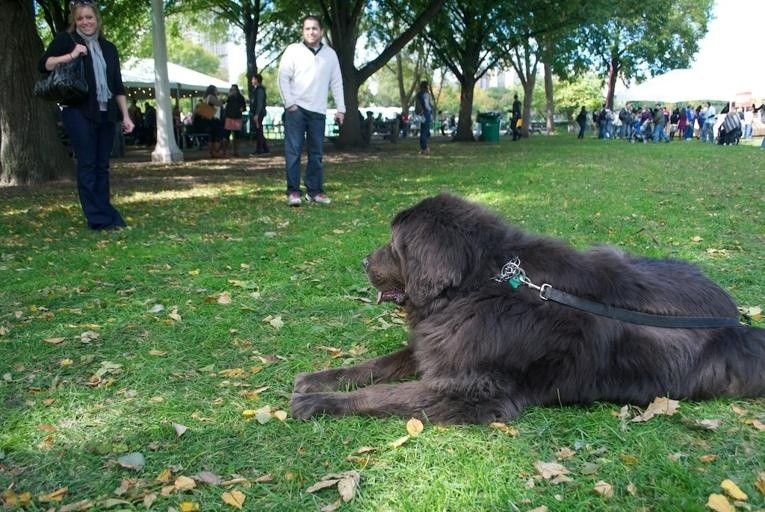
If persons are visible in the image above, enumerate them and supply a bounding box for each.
[277,15,346,207]
[56,74,268,156]
[334,80,523,156]
[576,101,759,147]
[37,1,136,233]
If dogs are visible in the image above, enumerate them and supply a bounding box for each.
[290,193,765,427]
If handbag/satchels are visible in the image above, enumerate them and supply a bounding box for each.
[226,117,241,129]
[413,115,424,122]
[35,56,88,102]
[197,102,216,120]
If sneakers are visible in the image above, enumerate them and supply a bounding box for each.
[304,192,331,204]
[423,147,429,153]
[288,191,300,205]
[249,150,271,158]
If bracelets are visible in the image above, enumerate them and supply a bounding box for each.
[70,52,75,60]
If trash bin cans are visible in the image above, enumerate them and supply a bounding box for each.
[477,112,502,142]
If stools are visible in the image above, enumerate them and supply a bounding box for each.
[122,120,235,149]
[333,121,542,137]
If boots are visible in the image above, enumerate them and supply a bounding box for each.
[208,138,242,159]
